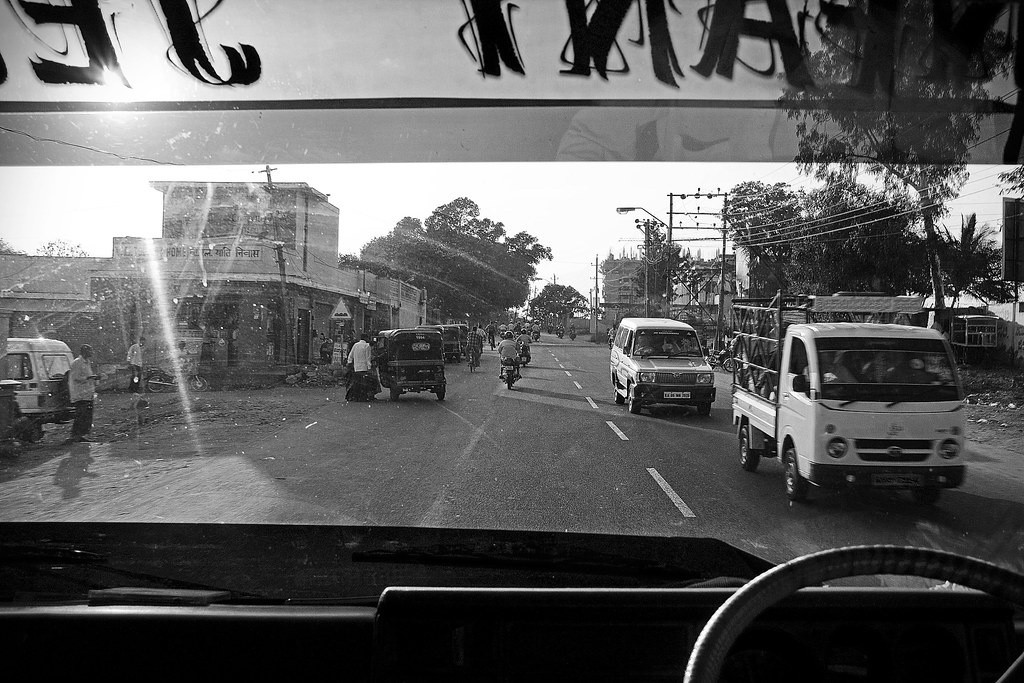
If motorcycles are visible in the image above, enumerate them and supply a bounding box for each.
[459,324,468,357]
[142,358,208,393]
[415,325,445,364]
[548,326,553,334]
[518,342,533,366]
[499,327,541,342]
[370,328,447,400]
[706,348,739,373]
[569,327,577,341]
[557,326,564,338]
[497,343,522,389]
[441,324,461,364]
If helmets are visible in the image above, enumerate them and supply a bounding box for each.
[521,328,526,334]
[505,331,514,339]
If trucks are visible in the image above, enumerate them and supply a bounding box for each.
[729,290,968,503]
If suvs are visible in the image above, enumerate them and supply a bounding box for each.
[7,337,75,424]
[609,317,716,415]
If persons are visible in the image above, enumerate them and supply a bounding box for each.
[516,328,533,365]
[344,334,386,403]
[608,326,617,348]
[548,321,553,333]
[485,321,496,348]
[802,352,856,382]
[464,326,483,367]
[167,341,188,360]
[127,338,146,392]
[635,335,653,354]
[494,320,541,339]
[884,354,940,383]
[320,339,333,363]
[477,325,486,353]
[557,321,565,336]
[569,322,576,337]
[67,344,101,441]
[498,331,523,379]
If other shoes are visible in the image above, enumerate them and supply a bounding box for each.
[78,435,95,442]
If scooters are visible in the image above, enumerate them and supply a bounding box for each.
[607,332,615,349]
[319,333,334,364]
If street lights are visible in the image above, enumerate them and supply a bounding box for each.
[616,206,670,320]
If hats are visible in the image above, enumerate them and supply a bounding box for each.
[471,326,477,331]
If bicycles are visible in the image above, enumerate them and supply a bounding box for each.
[485,330,498,350]
[466,339,484,373]
